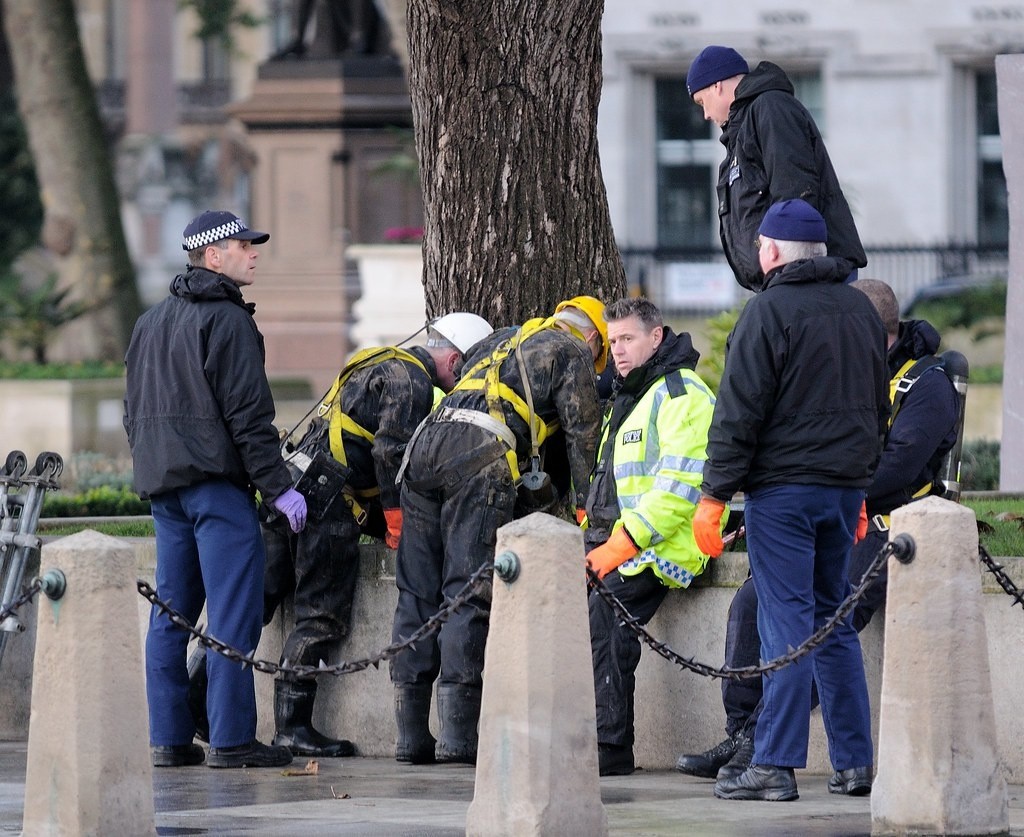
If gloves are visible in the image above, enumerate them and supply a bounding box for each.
[853,499,868,545]
[587,525,639,588]
[693,496,726,557]
[383,507,404,550]
[274,487,307,534]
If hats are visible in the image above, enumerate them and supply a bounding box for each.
[181,209,270,251]
[686,45,750,99]
[758,199,828,242]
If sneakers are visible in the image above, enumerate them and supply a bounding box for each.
[674,730,747,779]
[828,765,874,795]
[713,763,799,801]
[716,737,756,777]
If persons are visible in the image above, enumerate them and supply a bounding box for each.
[687,45,868,294]
[678,278,956,778]
[390,296,611,760]
[581,296,732,775]
[185,311,495,757]
[693,199,889,801]
[123,210,309,768]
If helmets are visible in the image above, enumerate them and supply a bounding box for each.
[555,295,611,374]
[428,312,496,355]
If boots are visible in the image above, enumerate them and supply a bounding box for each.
[185,646,211,744]
[271,676,356,757]
[434,684,482,763]
[393,683,437,763]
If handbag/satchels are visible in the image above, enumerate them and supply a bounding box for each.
[292,449,353,523]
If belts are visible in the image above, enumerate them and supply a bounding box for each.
[285,450,313,473]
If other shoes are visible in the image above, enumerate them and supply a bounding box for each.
[596,743,635,776]
[151,742,205,767]
[207,738,293,768]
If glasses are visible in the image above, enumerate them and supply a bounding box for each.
[754,239,762,250]
